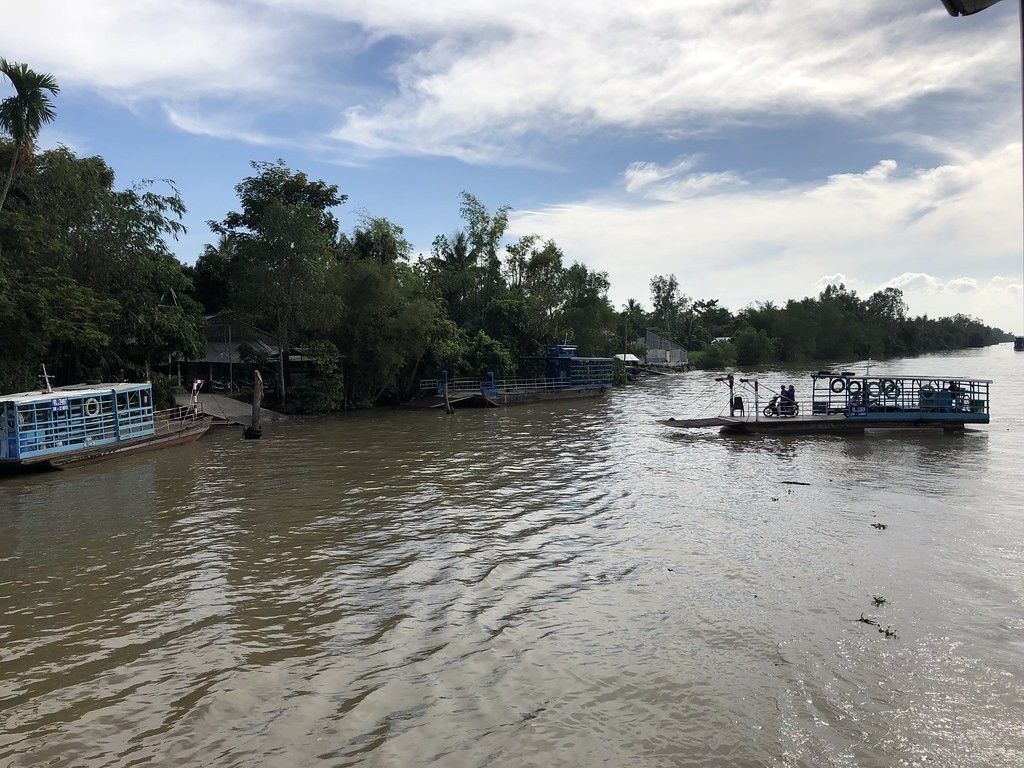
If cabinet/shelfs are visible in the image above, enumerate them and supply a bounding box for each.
[970,399,984,412]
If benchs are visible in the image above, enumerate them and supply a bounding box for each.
[812,402,828,415]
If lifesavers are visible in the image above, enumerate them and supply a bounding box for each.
[819,365,970,405]
[83,395,106,422]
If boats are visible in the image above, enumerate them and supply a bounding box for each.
[656,358,994,436]
[421,345,615,409]
[1014,338,1024,352]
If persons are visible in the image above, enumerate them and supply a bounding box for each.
[777,384,795,416]
[947,381,960,406]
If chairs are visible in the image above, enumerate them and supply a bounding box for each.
[733,397,744,416]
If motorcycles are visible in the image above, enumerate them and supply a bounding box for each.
[762,393,799,418]
